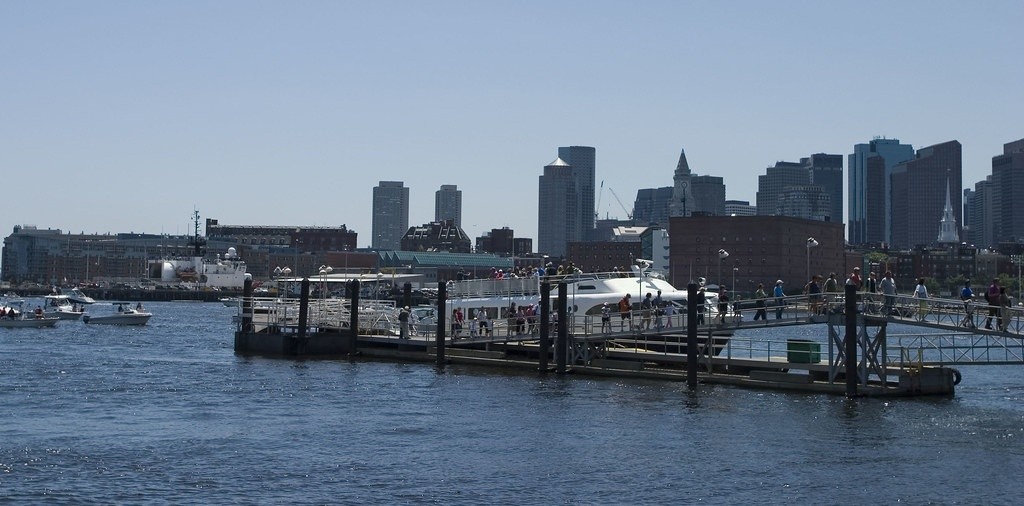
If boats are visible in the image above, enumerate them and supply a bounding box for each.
[0,290,25,307]
[51,287,95,304]
[38,292,90,320]
[83,301,152,325]
[423,248,744,357]
[218,297,261,308]
[0,305,60,327]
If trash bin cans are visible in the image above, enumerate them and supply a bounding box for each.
[787,338,821,363]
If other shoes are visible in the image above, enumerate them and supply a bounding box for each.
[985,326,992,330]
[922,316,928,322]
[961,319,966,327]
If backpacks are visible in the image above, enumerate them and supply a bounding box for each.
[960,289,964,301]
[984,290,990,302]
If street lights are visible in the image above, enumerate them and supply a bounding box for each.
[716,249,729,324]
[639,261,649,333]
[318,265,333,321]
[373,271,383,326]
[573,268,582,336]
[806,237,818,324]
[445,280,456,340]
[507,272,520,337]
[273,266,291,334]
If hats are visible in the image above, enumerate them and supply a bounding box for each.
[971,293,976,298]
[776,280,784,284]
[854,267,860,271]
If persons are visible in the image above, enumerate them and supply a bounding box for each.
[641,293,652,329]
[507,301,541,337]
[665,300,673,328]
[653,291,666,329]
[774,280,787,320]
[985,278,1011,332]
[601,302,614,334]
[733,295,744,323]
[612,266,629,278]
[457,267,470,283]
[1,308,6,317]
[620,294,632,332]
[913,278,929,322]
[399,306,411,339]
[878,271,897,318]
[137,303,141,310]
[805,273,837,315]
[35,305,42,319]
[450,307,465,341]
[844,267,861,313]
[713,285,729,323]
[698,287,707,325]
[489,261,583,280]
[754,283,768,321]
[960,278,976,328]
[80,305,84,312]
[865,272,876,314]
[118,304,122,312]
[8,307,15,320]
[477,306,489,336]
